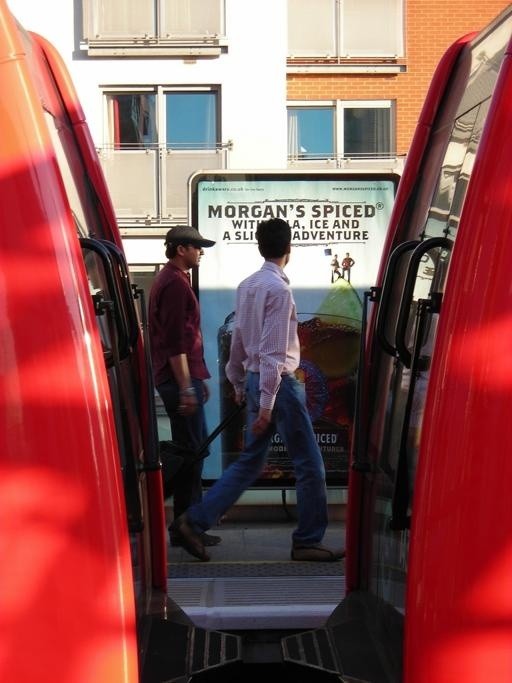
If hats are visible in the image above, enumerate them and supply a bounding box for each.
[166,226,215,247]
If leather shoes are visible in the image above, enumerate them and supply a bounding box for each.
[171,531,222,546]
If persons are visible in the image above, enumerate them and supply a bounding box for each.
[342,252,355,283]
[329,253,341,283]
[147,224,222,547]
[166,216,346,561]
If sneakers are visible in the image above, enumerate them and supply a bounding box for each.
[291,544,345,561]
[168,513,210,561]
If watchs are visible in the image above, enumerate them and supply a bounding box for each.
[179,387,196,395]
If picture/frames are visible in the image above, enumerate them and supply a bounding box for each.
[189,170,400,490]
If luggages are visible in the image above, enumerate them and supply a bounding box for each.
[159,399,247,486]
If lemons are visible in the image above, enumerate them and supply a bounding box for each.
[308,277,366,380]
